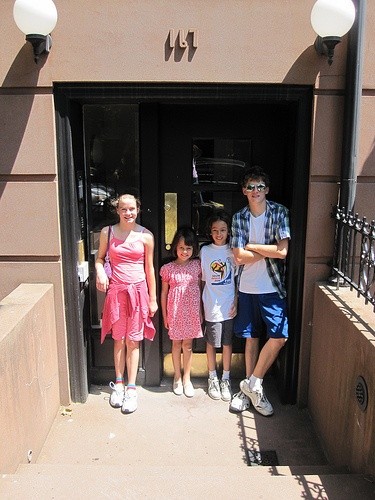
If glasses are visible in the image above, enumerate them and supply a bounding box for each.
[244,182,266,192]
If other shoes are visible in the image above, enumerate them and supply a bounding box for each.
[183,378,195,397]
[172,377,184,395]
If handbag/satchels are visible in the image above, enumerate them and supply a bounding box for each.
[104,225,113,279]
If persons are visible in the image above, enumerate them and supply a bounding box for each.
[96,196,158,413]
[230,166,291,416]
[197,216,239,402]
[160,229,205,397]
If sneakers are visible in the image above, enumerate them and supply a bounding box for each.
[207,377,220,400]
[229,389,250,412]
[108,381,126,407]
[220,379,234,401]
[239,378,274,416]
[122,386,138,413]
